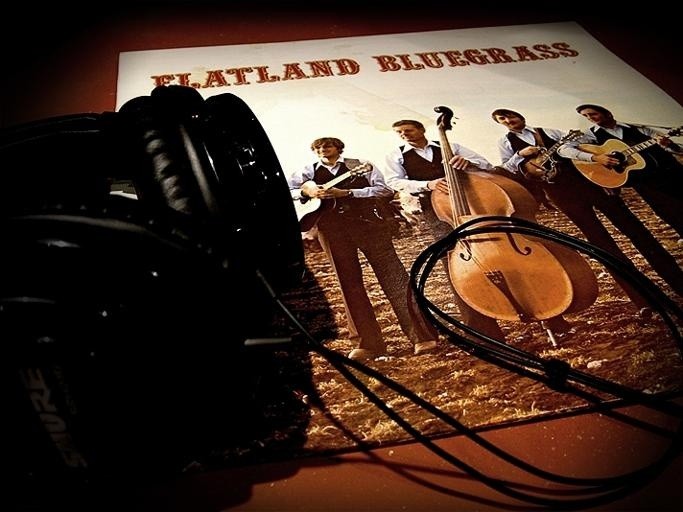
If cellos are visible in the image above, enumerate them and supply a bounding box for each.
[429,106,598,324]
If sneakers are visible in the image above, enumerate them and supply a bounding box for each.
[413,340,437,355]
[547,326,576,348]
[348,348,376,360]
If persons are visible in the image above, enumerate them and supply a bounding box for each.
[576,104,683,239]
[492,109,682,319]
[289,137,441,359]
[383,120,572,354]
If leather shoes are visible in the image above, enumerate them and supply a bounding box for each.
[638,306,653,318]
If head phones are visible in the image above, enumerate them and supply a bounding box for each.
[0,84,305,432]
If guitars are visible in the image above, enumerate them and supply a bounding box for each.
[572,126,682,189]
[517,129,584,182]
[289,163,373,230]
